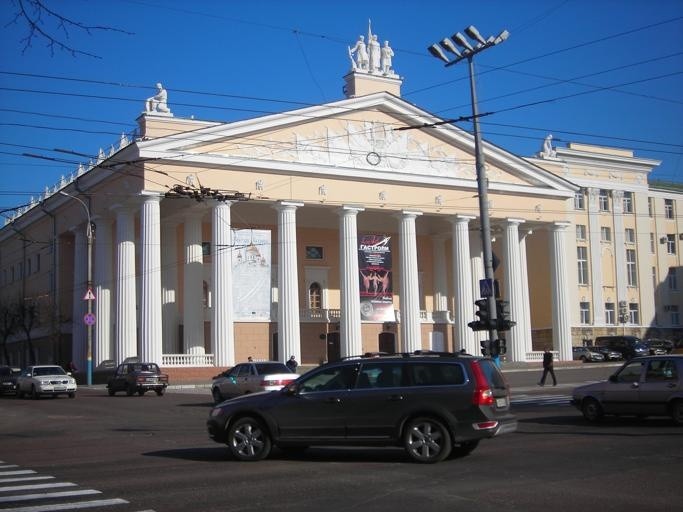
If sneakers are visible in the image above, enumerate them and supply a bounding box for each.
[553,383,557,386]
[537,383,544,386]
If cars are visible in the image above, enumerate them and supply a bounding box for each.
[570,355,683,423]
[104,361,172,399]
[210,357,301,405]
[569,333,674,364]
[0,361,80,400]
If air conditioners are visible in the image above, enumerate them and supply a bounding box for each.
[664,305,671,311]
[620,300,629,321]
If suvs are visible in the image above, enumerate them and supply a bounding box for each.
[207,344,515,466]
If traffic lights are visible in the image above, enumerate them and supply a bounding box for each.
[496,338,507,357]
[466,296,491,331]
[477,340,491,358]
[495,300,516,333]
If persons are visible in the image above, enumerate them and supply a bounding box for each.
[151,81,171,113]
[539,134,553,158]
[537,346,557,386]
[248,356,253,362]
[350,36,369,70]
[285,356,298,373]
[382,40,394,73]
[65,361,79,372]
[366,35,380,72]
[360,270,389,293]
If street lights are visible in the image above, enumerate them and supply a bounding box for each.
[422,20,515,380]
[55,190,96,385]
